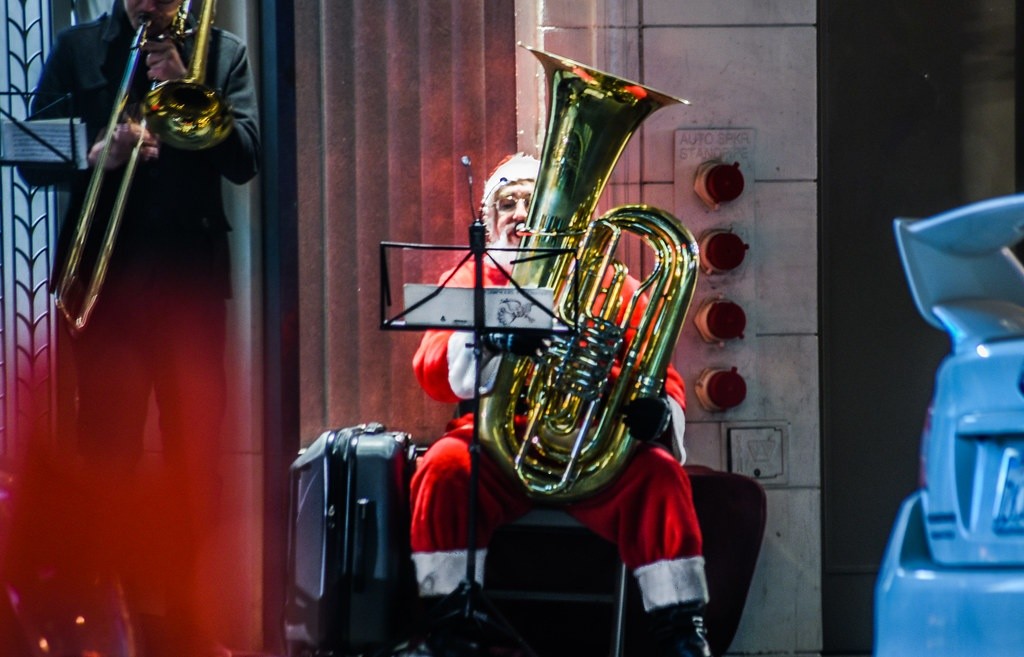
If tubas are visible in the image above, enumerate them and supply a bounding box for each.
[476,40,702,506]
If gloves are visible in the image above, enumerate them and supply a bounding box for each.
[617,395,672,441]
[483,330,552,357]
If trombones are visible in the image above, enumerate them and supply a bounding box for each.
[51,0,236,338]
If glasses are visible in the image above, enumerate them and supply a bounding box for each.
[491,196,531,213]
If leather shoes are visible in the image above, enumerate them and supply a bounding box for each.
[668,610,711,657]
[423,594,486,657]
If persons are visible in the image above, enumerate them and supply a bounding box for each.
[409,154,712,657]
[17,1,262,657]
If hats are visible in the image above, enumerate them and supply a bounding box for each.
[482,151,540,200]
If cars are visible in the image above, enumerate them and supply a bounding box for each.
[871,191,1024,655]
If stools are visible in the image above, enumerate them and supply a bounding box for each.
[489,462,769,657]
